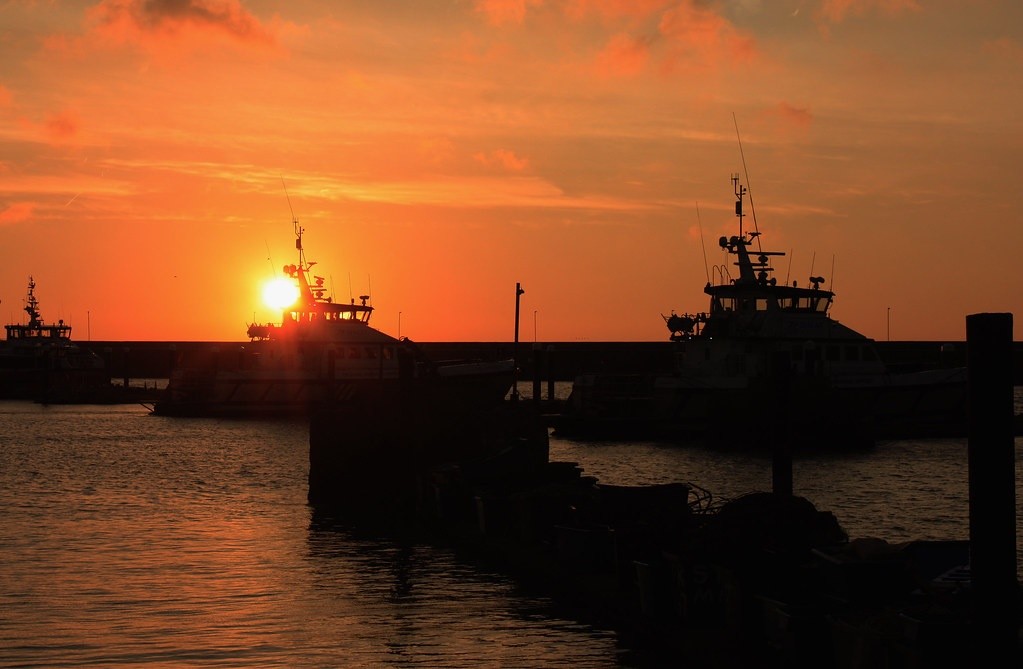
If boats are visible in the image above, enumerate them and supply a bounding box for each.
[0,278,107,404]
[544,173,984,454]
[142,216,520,423]
